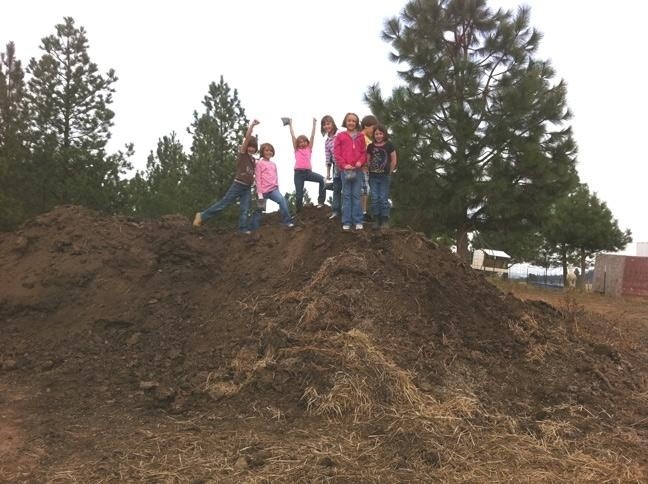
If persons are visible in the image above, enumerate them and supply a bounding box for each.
[319,115,342,220]
[366,123,396,230]
[333,113,368,231]
[193,118,260,235]
[288,117,326,212]
[359,115,376,215]
[251,142,293,233]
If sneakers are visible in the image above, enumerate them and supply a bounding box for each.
[363,212,389,229]
[343,225,351,230]
[356,224,364,230]
[317,203,337,219]
[192,212,202,227]
[245,223,295,235]
